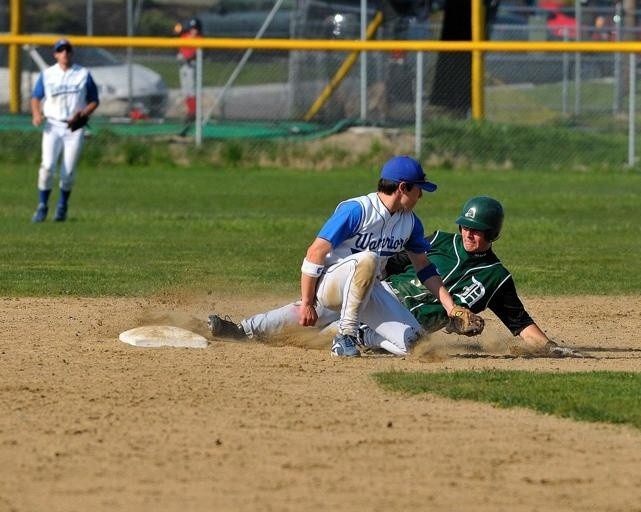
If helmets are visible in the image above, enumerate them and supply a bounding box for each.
[454,196,505,243]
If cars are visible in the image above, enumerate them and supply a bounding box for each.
[0,37,170,123]
[328,10,631,98]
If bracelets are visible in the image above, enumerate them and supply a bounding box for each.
[300,257,325,278]
[417,263,441,285]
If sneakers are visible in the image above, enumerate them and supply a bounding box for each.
[32,204,67,223]
[207,314,248,338]
[330,333,361,359]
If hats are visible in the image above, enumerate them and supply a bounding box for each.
[51,38,74,52]
[380,156,438,193]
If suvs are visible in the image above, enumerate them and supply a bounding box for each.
[181,0,387,66]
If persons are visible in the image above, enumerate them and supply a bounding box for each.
[300,155,485,358]
[172,17,202,123]
[208,195,596,359]
[29,37,99,223]
[387,47,415,104]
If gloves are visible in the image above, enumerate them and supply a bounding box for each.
[550,342,591,358]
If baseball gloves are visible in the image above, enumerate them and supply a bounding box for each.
[446,304,484,337]
